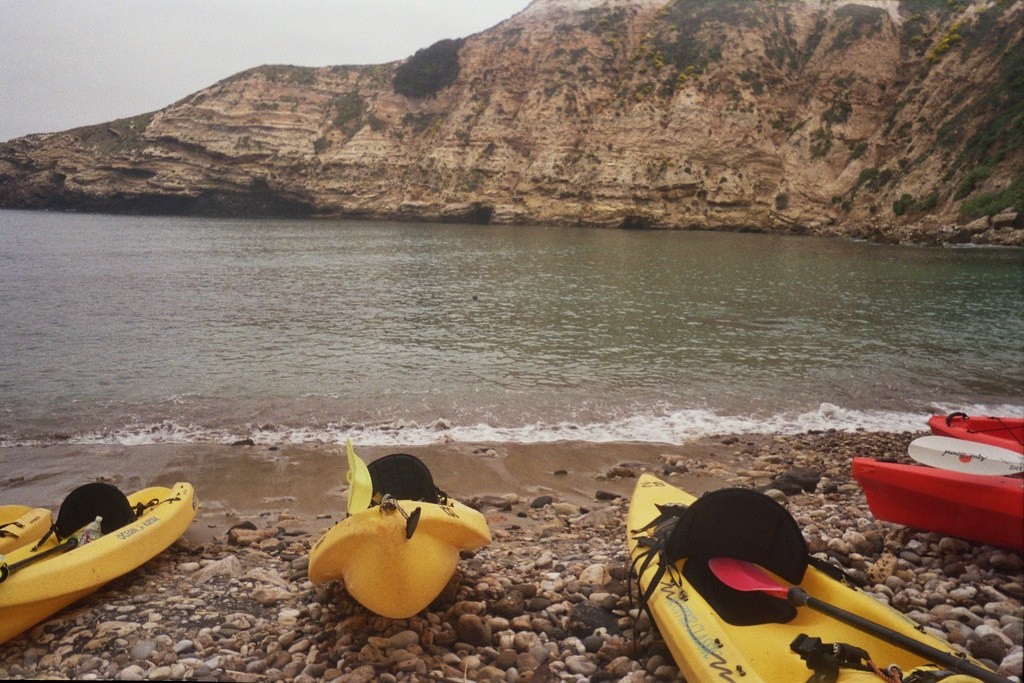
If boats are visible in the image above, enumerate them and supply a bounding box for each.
[848,410,1023,556]
[305,453,495,621]
[626,473,1001,683]
[4,482,199,649]
[1,505,54,562]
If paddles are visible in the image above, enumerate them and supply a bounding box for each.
[707,556,1016,683]
[0,537,79,584]
[908,436,1024,475]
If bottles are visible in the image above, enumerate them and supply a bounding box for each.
[75,515,103,548]
